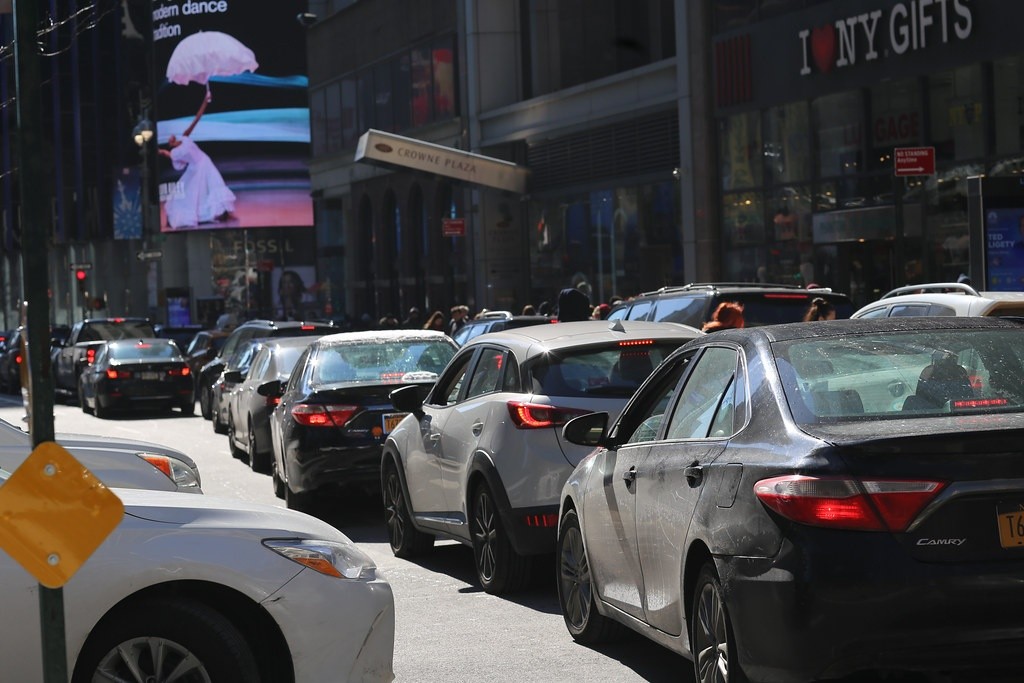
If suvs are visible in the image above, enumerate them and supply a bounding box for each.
[847,280,1024,328]
[451,307,557,350]
[601,280,859,335]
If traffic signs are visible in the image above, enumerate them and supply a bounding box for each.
[894,146,936,177]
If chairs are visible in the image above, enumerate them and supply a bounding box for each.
[803,390,864,417]
[611,352,658,388]
[902,364,974,411]
[404,345,450,372]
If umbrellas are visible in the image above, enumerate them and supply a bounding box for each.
[165,30,258,102]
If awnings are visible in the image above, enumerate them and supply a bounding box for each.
[353,128,525,197]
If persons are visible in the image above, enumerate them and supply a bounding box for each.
[805,298,835,321]
[157,94,238,226]
[363,305,472,336]
[279,271,313,305]
[259,310,317,321]
[523,283,622,322]
[704,302,743,333]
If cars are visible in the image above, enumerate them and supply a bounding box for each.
[0,468,395,683]
[0,315,211,407]
[553,314,1024,683]
[175,317,379,473]
[0,415,203,496]
[256,328,464,519]
[76,337,198,418]
[380,319,711,598]
